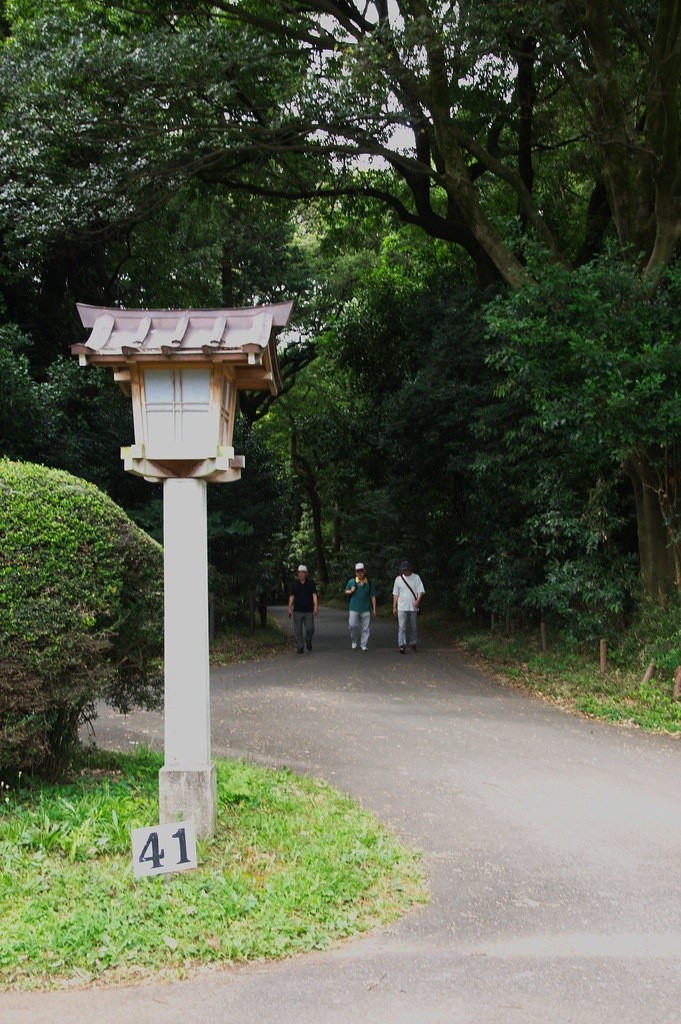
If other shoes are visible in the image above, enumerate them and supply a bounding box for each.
[297,647,303,653]
[352,643,357,649]
[400,646,404,652]
[307,642,312,650]
[362,647,368,650]
[410,645,417,652]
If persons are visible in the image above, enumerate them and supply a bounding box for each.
[345,563,376,651]
[392,561,425,654]
[288,565,318,655]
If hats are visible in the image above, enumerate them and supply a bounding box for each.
[298,565,307,572]
[355,563,364,570]
[401,561,410,570]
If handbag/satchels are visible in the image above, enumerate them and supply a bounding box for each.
[417,604,422,615]
